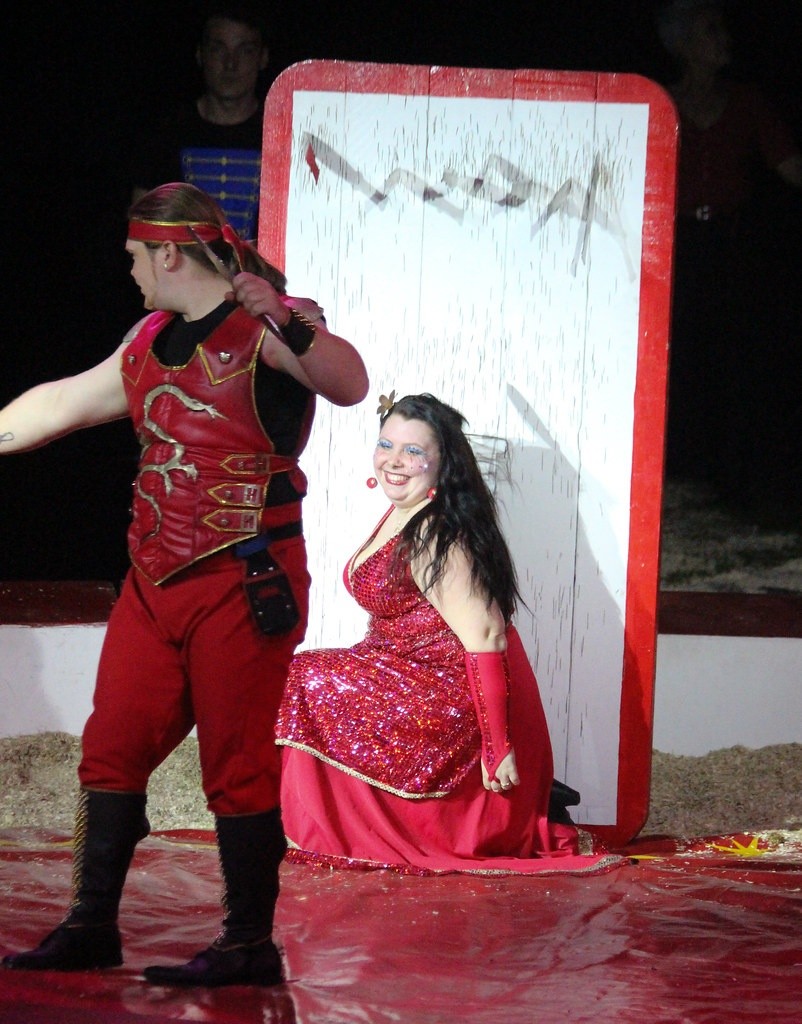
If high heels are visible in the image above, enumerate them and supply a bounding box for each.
[548,778,580,824]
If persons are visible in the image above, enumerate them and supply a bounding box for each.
[130,18,265,245]
[655,0,802,497]
[275,391,632,879]
[0,182,368,990]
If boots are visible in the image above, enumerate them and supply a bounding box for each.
[2,787,150,970]
[143,805,289,988]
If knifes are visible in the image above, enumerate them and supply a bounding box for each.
[189,224,282,339]
